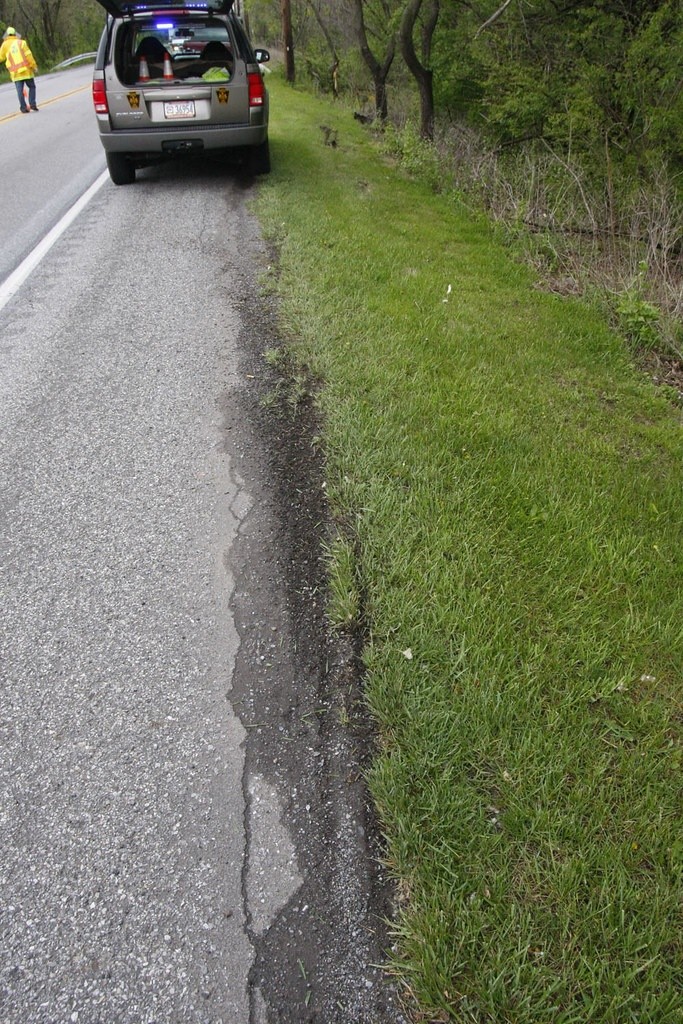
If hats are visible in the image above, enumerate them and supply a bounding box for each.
[7,27,15,34]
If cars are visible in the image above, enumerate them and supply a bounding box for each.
[168,29,234,55]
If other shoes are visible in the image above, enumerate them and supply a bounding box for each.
[21,108,28,112]
[31,106,39,112]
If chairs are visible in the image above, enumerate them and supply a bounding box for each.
[201,41,233,65]
[134,37,175,64]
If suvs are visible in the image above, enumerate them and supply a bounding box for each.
[92,0,272,186]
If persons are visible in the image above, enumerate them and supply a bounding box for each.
[0,26,41,112]
